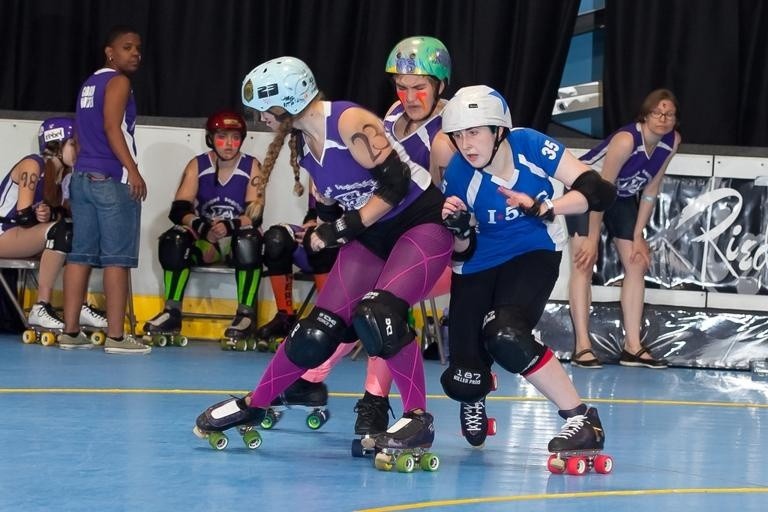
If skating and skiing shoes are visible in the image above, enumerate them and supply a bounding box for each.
[194,391,268,450]
[260,377,330,429]
[460,374,497,446]
[351,390,395,457]
[374,408,439,472]
[546,403,612,475]
[23,301,301,354]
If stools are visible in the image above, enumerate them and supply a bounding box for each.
[177,262,240,321]
[348,297,448,367]
[286,263,322,321]
[0,258,59,338]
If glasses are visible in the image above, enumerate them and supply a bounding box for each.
[651,111,676,119]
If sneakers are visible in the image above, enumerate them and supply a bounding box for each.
[571,349,603,369]
[620,347,668,368]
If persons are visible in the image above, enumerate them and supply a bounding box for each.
[0,116,109,329]
[59,25,152,353]
[141,112,266,339]
[564,89,682,368]
[270,37,453,434]
[438,83,605,452]
[196,56,456,451]
[259,174,341,337]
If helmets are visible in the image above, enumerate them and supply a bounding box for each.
[38,117,75,157]
[385,37,452,85]
[441,84,512,133]
[205,108,246,133]
[241,56,319,114]
